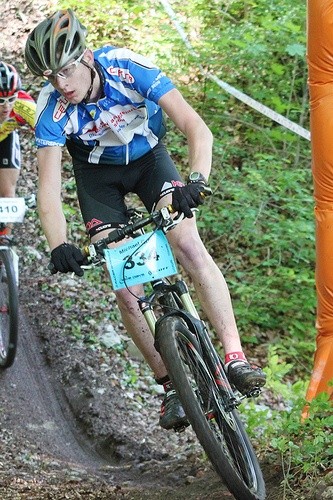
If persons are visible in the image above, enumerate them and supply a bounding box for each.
[25,9,266,429]
[0,61,38,236]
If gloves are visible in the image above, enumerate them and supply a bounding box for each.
[171,180,214,218]
[50,243,92,277]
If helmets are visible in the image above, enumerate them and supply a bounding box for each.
[0,61,22,97]
[25,7,88,77]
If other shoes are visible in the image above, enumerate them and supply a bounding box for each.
[1,258,8,281]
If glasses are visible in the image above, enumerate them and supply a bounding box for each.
[43,47,87,83]
[0,92,18,105]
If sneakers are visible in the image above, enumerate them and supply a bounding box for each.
[223,358,266,398]
[159,394,187,430]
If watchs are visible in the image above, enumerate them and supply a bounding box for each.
[186,171,209,186]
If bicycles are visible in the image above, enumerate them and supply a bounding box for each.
[48,184,267,500]
[0,194,38,370]
